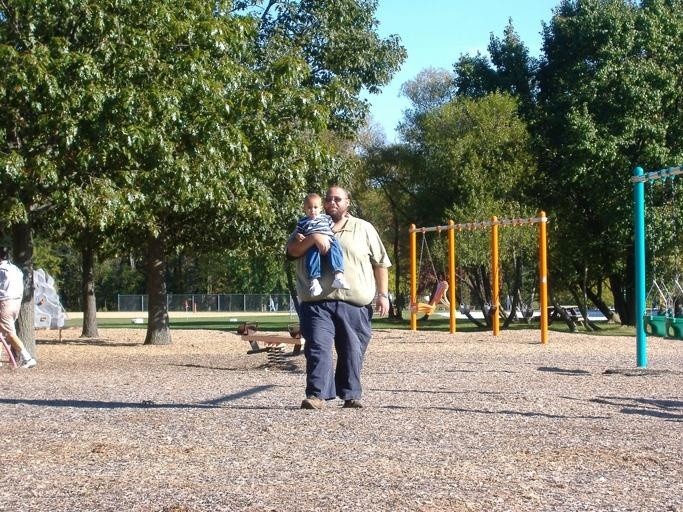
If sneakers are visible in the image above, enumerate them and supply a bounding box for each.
[300,394,326,409]
[331,278,349,290]
[308,284,323,297]
[344,400,362,408]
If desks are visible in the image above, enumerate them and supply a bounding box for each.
[536,305,585,327]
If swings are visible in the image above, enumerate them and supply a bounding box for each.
[416,223,540,323]
[644,178,683,338]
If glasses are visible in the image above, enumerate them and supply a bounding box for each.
[325,197,340,202]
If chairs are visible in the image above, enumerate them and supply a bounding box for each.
[409,281,448,315]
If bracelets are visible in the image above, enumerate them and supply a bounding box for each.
[377,291,389,299]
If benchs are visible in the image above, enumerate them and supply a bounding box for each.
[567,315,583,322]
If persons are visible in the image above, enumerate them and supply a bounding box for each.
[293,192,350,298]
[0,246,37,368]
[285,185,390,409]
